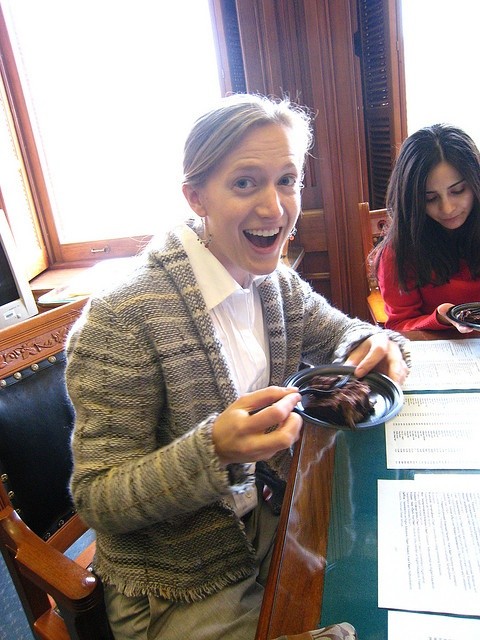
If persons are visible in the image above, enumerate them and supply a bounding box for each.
[372,122,480,335]
[62,92,414,640]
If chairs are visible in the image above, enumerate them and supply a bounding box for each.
[357,200,401,326]
[1,295,107,640]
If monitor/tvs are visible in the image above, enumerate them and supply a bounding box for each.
[0,209,39,330]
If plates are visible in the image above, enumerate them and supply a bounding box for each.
[283,365,405,429]
[447,301,480,328]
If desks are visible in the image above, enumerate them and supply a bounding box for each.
[255,328,478,639]
